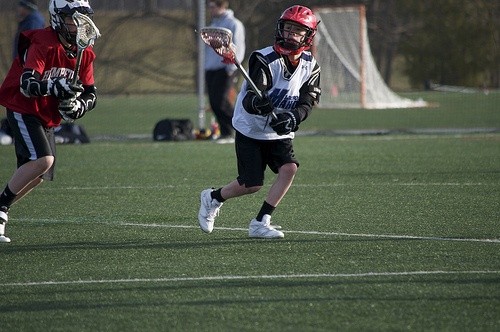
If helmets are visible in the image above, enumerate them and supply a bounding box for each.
[47,0,95,33]
[272,4,318,54]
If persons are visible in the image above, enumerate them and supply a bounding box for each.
[198,5,320,239]
[203,0,245,138]
[13,0,45,58]
[0,0,98,243]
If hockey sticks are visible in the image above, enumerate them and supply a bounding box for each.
[199,26,280,122]
[71,12,102,91]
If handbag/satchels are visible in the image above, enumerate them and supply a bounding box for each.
[152,118,194,141]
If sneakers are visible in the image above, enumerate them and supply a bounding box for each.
[248,214,285,238]
[198,187,223,234]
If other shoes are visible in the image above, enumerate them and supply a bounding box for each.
[0,205,8,223]
[0,221,9,243]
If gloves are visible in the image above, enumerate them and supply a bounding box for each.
[254,93,276,118]
[47,76,86,100]
[269,111,294,135]
[57,96,87,120]
